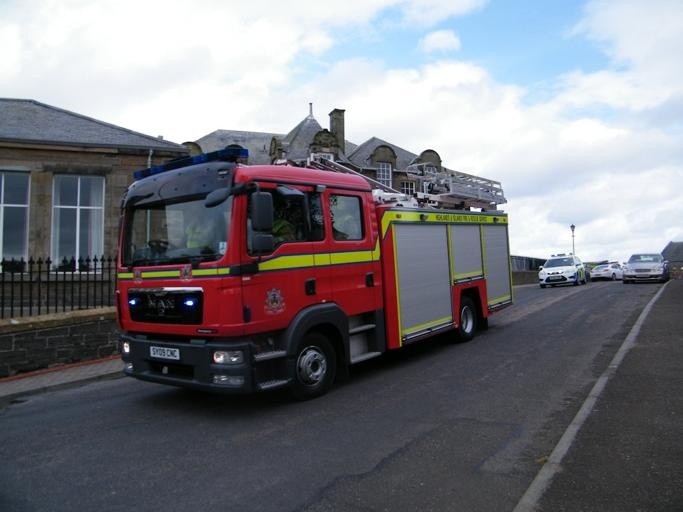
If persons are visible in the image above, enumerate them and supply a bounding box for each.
[329,197,355,242]
[155,202,223,250]
[248,196,297,245]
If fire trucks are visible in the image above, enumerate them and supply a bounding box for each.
[114,143,520,404]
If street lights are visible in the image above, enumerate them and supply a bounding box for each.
[569,224,576,255]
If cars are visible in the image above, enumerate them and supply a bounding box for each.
[536,251,670,289]
